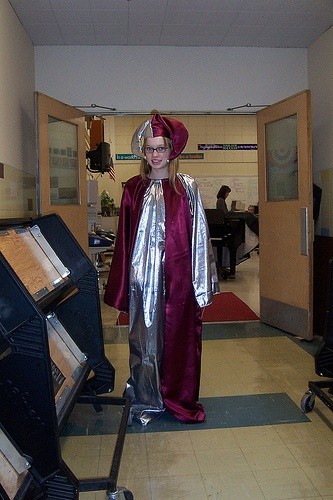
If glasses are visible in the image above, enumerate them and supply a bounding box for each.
[144,147,170,153]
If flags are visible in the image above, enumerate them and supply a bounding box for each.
[107,157,116,183]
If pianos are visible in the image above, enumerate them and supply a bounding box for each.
[204,208,254,281]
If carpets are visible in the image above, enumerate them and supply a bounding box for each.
[115,292,260,326]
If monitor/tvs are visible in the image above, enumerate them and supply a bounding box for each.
[89,142,111,174]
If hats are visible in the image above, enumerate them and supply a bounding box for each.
[131,113,189,162]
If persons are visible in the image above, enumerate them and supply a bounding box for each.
[101,114,220,428]
[215,184,231,214]
[247,205,254,215]
